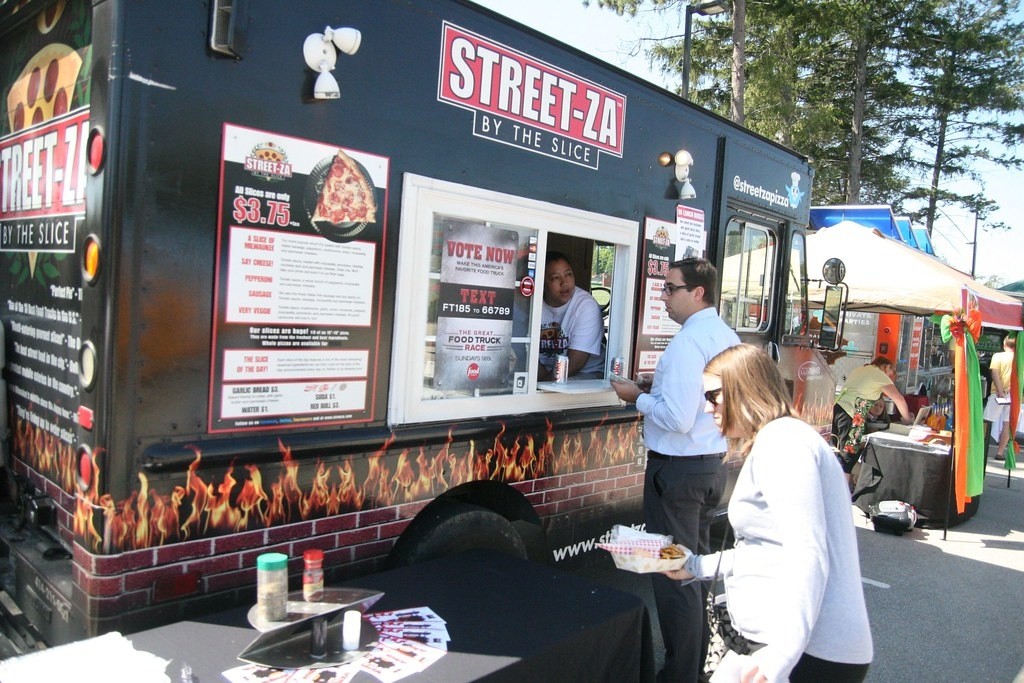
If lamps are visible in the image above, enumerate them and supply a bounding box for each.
[313,57,343,101]
[672,149,695,168]
[322,25,362,56]
[680,178,696,202]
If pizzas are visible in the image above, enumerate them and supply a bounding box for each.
[310,148,376,223]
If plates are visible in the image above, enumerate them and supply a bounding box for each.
[303,154,378,243]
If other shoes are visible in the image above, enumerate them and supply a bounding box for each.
[995,454,1005,460]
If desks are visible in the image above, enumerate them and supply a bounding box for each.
[852,429,979,527]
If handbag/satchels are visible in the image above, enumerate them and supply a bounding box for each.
[704,604,765,678]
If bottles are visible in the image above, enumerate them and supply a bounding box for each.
[257,553,288,622]
[301,549,326,601]
[925,402,949,431]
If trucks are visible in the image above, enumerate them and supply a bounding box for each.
[0,0,850,660]
[786,300,953,408]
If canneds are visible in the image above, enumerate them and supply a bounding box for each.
[552,354,569,383]
[256,549,324,622]
[609,355,624,379]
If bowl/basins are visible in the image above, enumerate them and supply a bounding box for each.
[604,525,692,573]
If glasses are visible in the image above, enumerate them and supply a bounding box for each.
[704,387,723,406]
[661,285,690,294]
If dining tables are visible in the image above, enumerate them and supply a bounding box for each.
[2,533,693,682]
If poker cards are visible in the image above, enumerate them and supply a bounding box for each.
[221,605,452,683]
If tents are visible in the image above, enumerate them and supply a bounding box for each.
[709,218,1024,546]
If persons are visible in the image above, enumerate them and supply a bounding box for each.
[539,248,610,380]
[508,252,531,377]
[603,256,752,683]
[981,335,1018,460]
[650,343,876,683]
[862,395,893,437]
[825,354,915,499]
